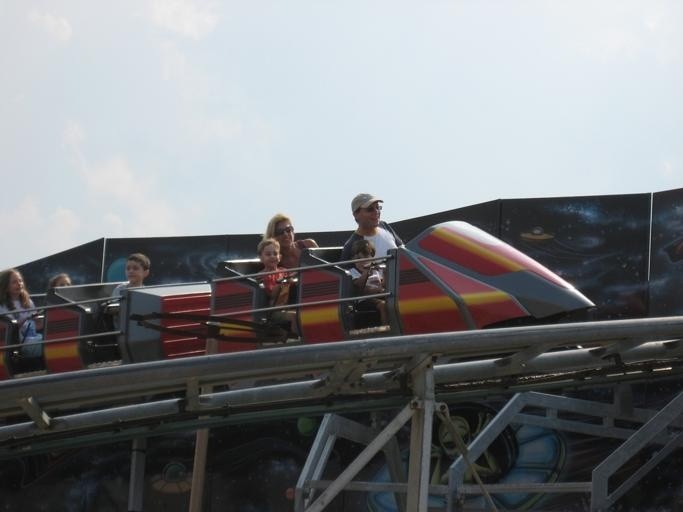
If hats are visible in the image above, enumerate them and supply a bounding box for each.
[352,193,383,214]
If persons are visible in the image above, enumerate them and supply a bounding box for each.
[264,215,319,270]
[109,253,152,329]
[342,191,396,263]
[47,272,71,291]
[256,237,299,337]
[0,268,44,355]
[344,241,389,327]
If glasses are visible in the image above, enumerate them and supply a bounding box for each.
[273,226,293,236]
[367,206,382,212]
